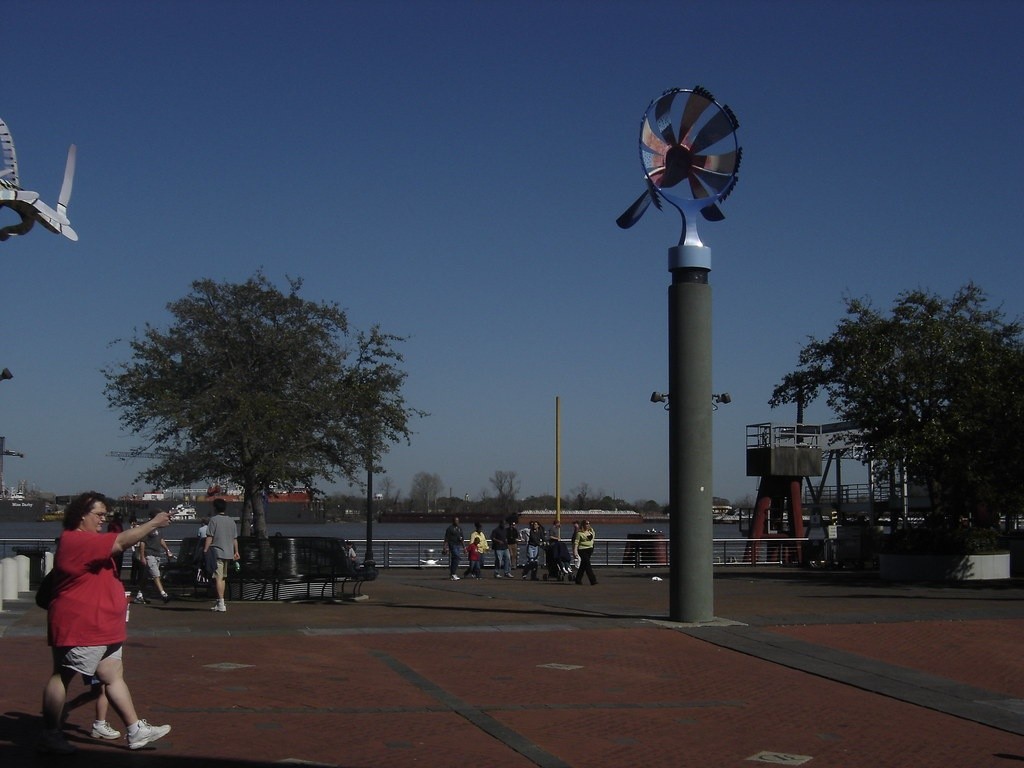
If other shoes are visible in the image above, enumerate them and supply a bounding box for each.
[161,594,173,602]
[591,580,598,584]
[532,576,538,580]
[210,603,226,612]
[463,572,466,577]
[504,572,514,577]
[522,573,528,580]
[450,573,460,581]
[576,580,582,584]
[494,573,502,578]
[476,576,483,580]
[134,597,150,604]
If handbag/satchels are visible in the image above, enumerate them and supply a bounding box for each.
[36,565,54,609]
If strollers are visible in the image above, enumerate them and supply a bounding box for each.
[537,540,577,583]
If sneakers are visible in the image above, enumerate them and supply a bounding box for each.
[92,723,121,740]
[37,731,76,754]
[125,716,170,750]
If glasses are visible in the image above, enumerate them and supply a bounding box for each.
[88,510,108,519]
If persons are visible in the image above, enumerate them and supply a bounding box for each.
[490,520,514,579]
[519,521,534,547]
[37,491,178,750]
[204,499,240,611]
[523,521,546,581]
[198,519,209,540]
[464,537,484,580]
[107,511,124,578]
[574,519,598,585]
[131,508,173,604]
[470,522,489,569]
[549,520,560,544]
[129,516,144,585]
[505,521,519,570]
[572,521,581,570]
[443,516,467,581]
[344,542,356,561]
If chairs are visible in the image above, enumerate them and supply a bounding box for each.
[159,537,379,601]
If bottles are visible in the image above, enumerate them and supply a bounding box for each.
[234,557,241,572]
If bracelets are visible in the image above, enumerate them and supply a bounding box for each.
[168,549,169,552]
